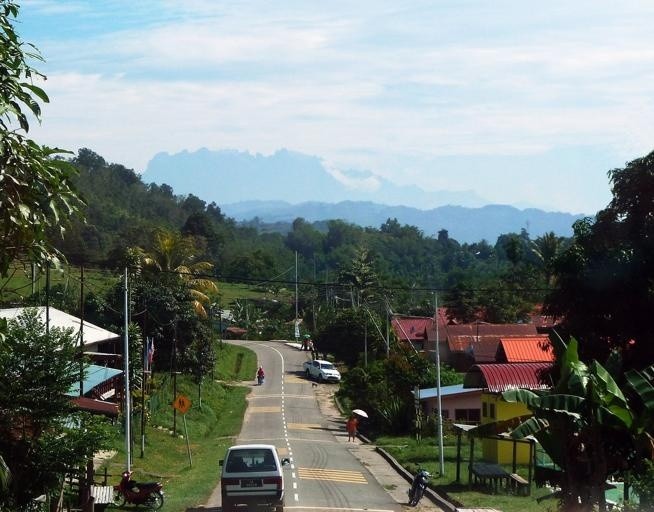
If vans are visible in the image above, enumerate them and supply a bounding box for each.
[219,445,290,512]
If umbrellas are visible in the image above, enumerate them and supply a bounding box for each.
[352,409,368,419]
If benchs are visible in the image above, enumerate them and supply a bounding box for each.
[468,464,530,495]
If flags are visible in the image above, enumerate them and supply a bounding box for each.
[148,340,156,364]
[144,339,149,370]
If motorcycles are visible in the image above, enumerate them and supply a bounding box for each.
[406,462,439,508]
[111,471,165,511]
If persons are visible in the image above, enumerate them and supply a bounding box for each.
[345,415,359,442]
[301,337,313,350]
[257,366,265,383]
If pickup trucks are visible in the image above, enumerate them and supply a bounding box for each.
[303,360,341,384]
